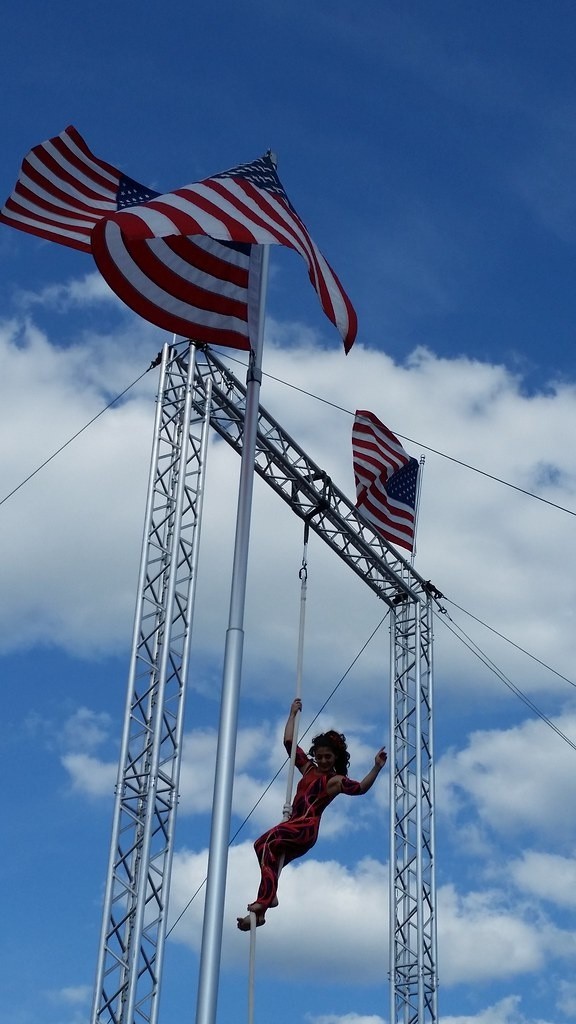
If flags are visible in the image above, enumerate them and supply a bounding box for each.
[350,408,423,555]
[85,150,361,359]
[1,124,169,256]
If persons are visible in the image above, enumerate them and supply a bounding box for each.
[234,696,388,932]
